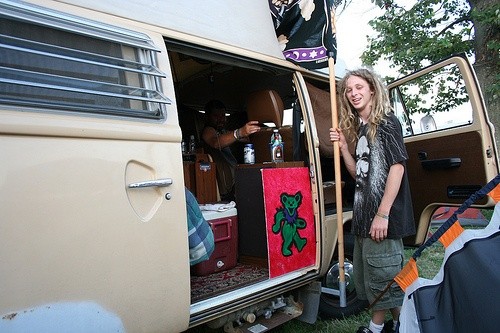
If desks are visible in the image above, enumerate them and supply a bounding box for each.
[235,161,304,266]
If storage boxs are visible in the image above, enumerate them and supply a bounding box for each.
[191,208,239,277]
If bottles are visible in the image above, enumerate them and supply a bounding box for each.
[270,129,284,163]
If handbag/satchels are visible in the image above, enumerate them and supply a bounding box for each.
[211,134,239,198]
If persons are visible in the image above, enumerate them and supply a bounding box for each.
[330,69,417,333]
[192,100,261,201]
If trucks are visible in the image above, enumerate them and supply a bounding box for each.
[0,0,500,332]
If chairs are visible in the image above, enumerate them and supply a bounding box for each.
[179,106,240,193]
[247,89,345,205]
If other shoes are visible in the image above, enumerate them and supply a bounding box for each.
[384,319,400,333]
[356,326,389,333]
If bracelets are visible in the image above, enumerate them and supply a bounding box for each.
[234,129,242,140]
[377,212,388,218]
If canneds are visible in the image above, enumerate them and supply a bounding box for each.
[244,144,255,164]
